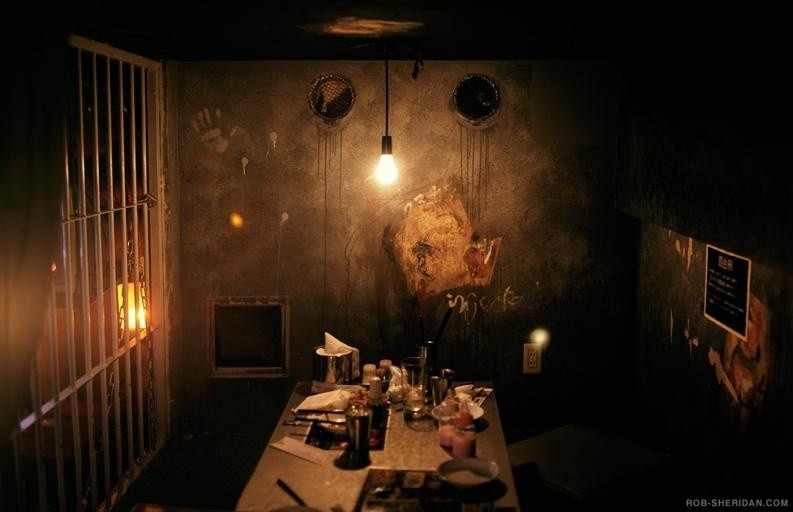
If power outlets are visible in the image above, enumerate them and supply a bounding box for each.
[522,342,543,375]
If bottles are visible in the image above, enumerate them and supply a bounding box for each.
[364,376,389,449]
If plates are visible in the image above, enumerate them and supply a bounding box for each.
[430,401,485,421]
[435,457,501,486]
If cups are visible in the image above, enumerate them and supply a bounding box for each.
[400,356,427,419]
[343,404,373,469]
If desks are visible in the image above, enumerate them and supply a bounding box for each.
[235,380,519,512]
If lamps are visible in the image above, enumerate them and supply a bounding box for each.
[119,282,148,331]
[374,60,398,186]
